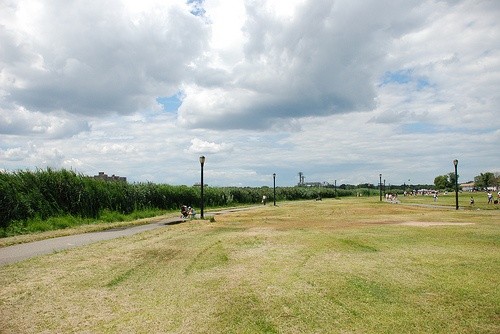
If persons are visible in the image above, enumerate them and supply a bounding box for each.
[487,190,493,204]
[434,193,437,200]
[386,192,395,201]
[470,196,474,206]
[497,190,500,203]
[188,207,196,218]
[262,194,266,205]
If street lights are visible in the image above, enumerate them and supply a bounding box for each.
[272,173,276,205]
[199,155,206,220]
[379,173,382,201]
[452,159,459,210]
